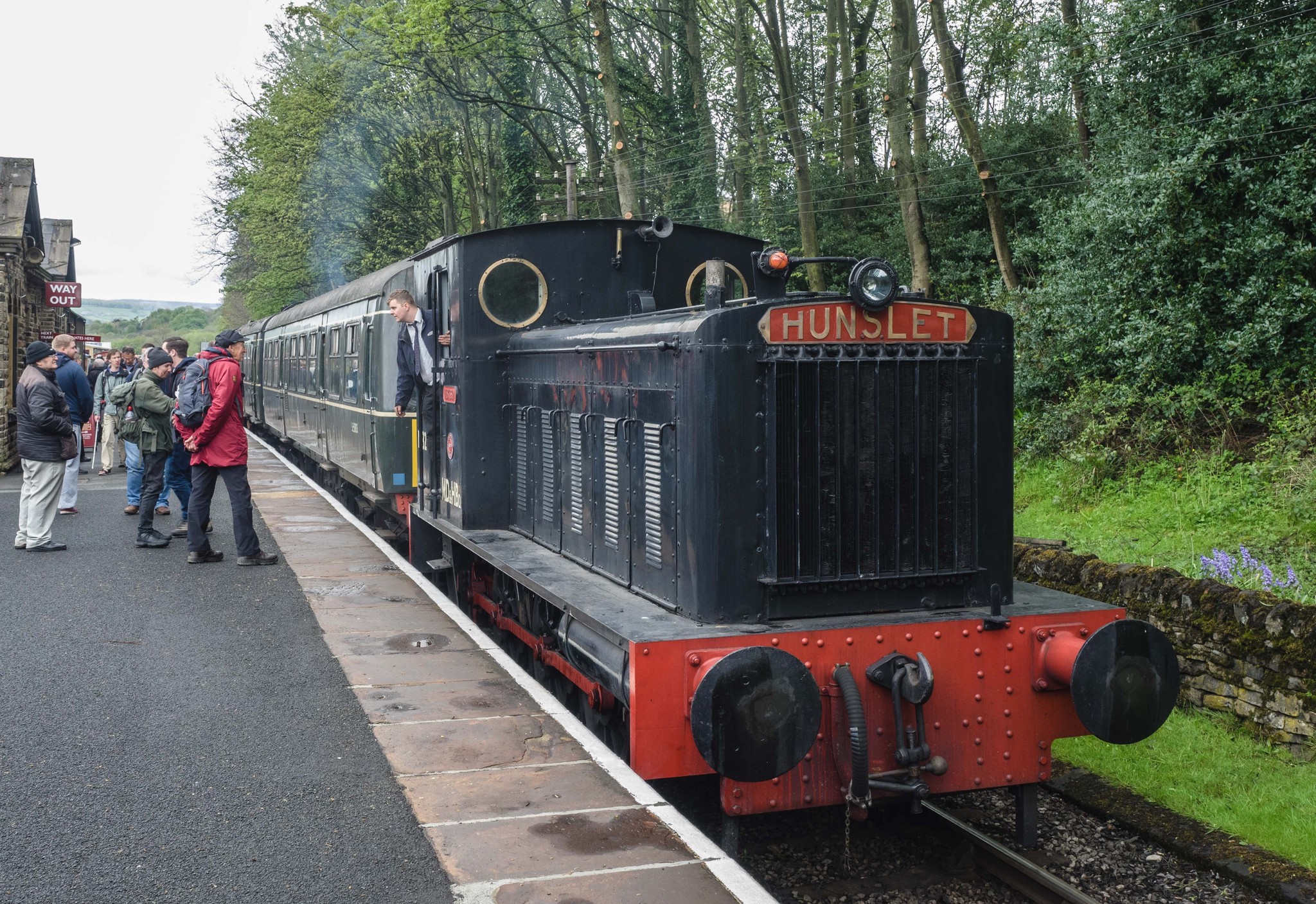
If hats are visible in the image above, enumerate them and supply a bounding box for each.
[26,341,56,364]
[147,347,174,370]
[214,330,250,348]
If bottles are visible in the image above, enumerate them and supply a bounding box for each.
[125,406,136,422]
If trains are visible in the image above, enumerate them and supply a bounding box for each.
[232,212,1183,874]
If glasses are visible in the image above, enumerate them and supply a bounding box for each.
[236,343,246,348]
[123,358,133,362]
[49,353,58,360]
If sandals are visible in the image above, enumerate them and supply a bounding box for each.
[98,469,111,475]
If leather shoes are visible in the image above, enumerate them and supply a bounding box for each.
[187,548,223,563]
[26,540,67,551]
[236,552,279,566]
[15,545,26,549]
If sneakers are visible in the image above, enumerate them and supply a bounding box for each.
[150,528,172,540]
[136,533,169,548]
[124,505,139,515]
[156,507,170,515]
[171,517,213,538]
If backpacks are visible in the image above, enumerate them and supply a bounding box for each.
[108,377,149,443]
[175,356,229,427]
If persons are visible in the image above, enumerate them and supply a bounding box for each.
[14,340,79,552]
[72,343,173,516]
[135,347,179,548]
[52,334,94,514]
[292,359,317,392]
[386,289,450,482]
[162,338,213,537]
[346,358,357,399]
[174,330,279,565]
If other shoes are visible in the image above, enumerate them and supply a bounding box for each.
[60,507,78,514]
[80,457,91,462]
[79,469,88,474]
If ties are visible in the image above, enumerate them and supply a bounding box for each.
[409,321,421,376]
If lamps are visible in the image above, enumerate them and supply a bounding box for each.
[70,238,81,247]
[68,322,75,330]
[22,235,44,263]
